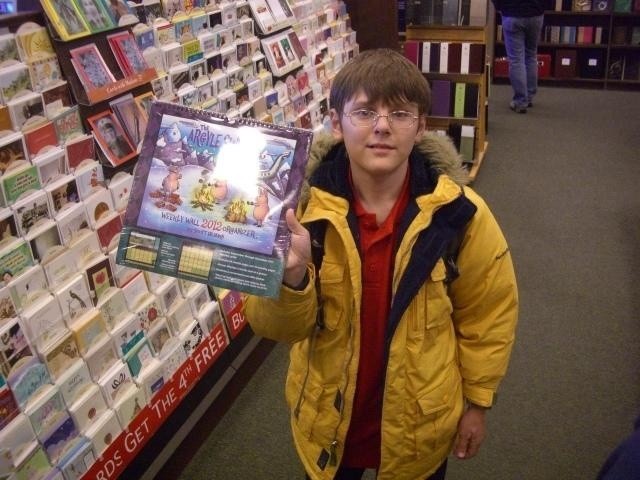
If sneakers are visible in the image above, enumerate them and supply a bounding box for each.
[510,97,534,115]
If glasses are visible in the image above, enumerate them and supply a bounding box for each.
[336,104,424,130]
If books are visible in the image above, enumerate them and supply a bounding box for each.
[1,0,359,479]
[494,2,640,86]
[406,34,489,176]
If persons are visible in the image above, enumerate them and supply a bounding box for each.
[491,1,551,114]
[240,48,521,480]
